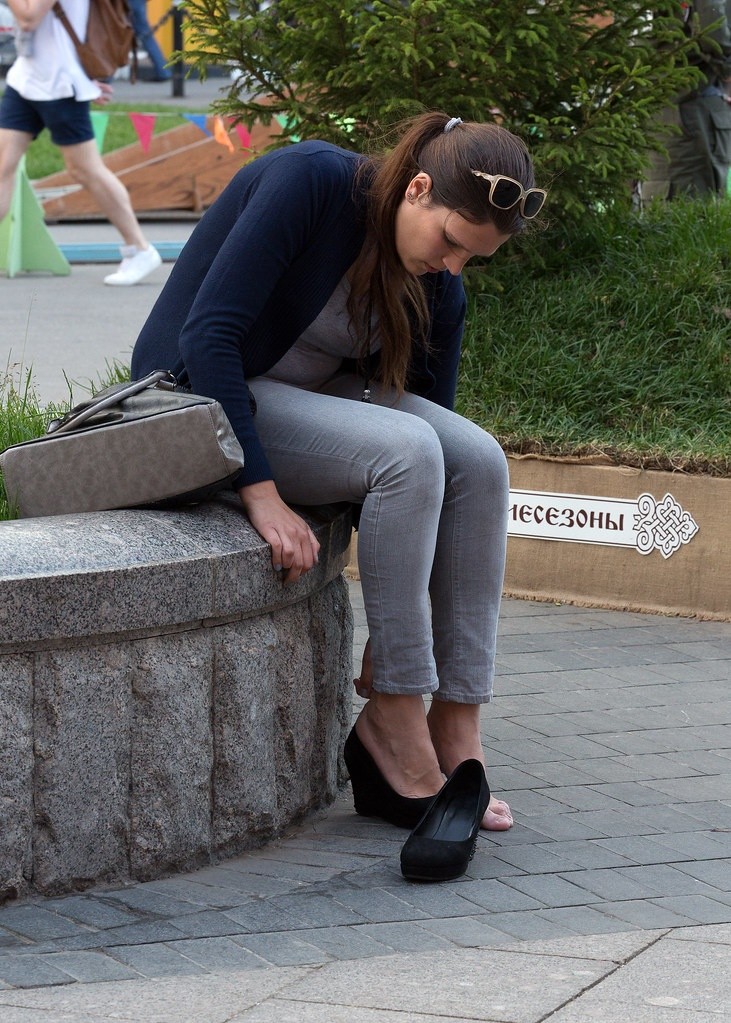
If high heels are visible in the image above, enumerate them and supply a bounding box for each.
[344,724,439,830]
[400,758,490,882]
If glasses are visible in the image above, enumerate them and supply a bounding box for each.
[471,170,547,220]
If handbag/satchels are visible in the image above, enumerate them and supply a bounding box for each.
[0,369,244,519]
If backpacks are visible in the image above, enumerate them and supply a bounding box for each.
[49,0,134,81]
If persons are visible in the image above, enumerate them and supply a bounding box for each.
[103,1,173,82]
[633,0,731,205]
[0,0,162,287]
[129,112,550,831]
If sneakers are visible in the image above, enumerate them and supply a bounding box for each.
[104,245,162,284]
